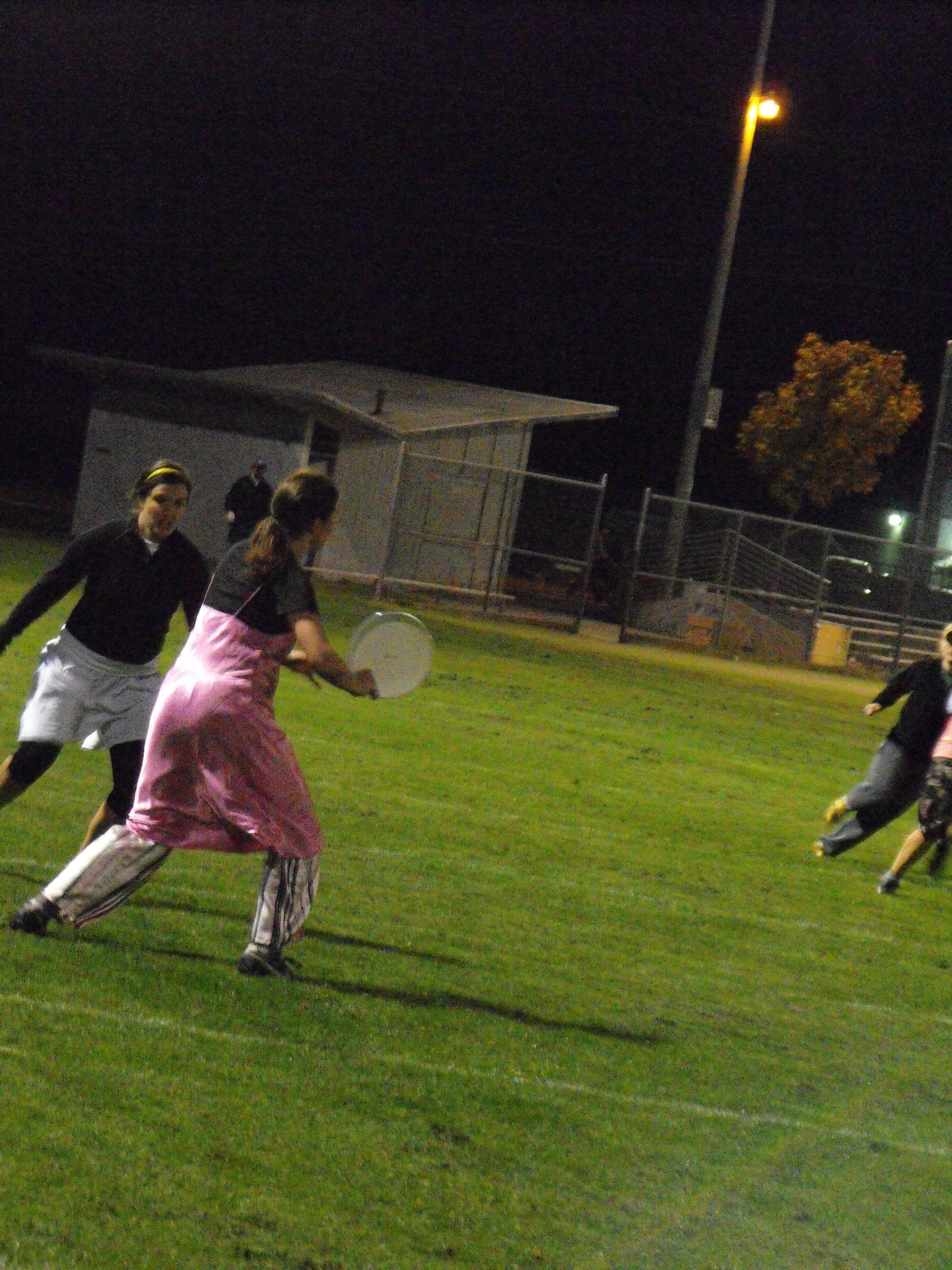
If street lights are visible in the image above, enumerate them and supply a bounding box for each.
[654,0,783,600]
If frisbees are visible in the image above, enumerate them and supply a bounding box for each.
[345,609,433,701]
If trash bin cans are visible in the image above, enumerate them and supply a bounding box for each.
[807,618,855,667]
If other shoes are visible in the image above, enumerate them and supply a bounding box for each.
[825,794,849,824]
[876,874,902,896]
[10,909,50,938]
[237,942,303,981]
[813,838,824,860]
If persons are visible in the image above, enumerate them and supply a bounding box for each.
[0,460,211,853]
[225,458,275,548]
[812,622,952,894]
[9,470,380,985]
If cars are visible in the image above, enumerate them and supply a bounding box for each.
[826,554,951,620]
